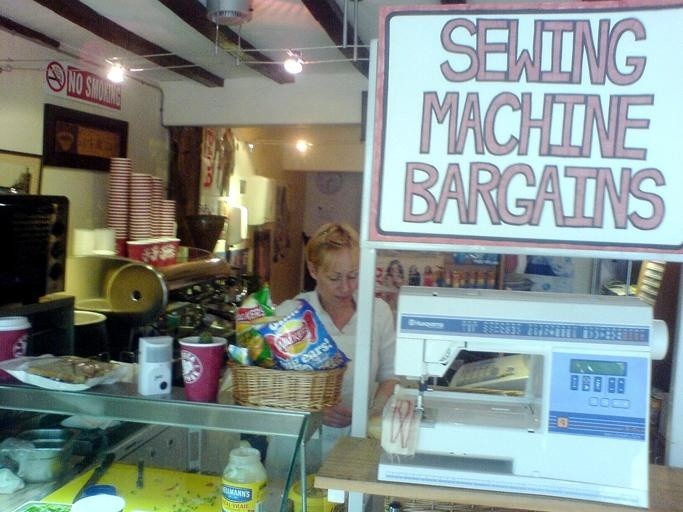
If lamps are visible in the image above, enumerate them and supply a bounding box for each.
[284,45,303,75]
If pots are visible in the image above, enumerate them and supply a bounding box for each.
[73,310,107,355]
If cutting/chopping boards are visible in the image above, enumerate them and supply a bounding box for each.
[43,456,271,512]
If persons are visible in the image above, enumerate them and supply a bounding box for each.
[273,221,395,466]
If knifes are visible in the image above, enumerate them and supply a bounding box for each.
[73,451,122,506]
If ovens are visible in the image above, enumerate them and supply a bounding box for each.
[0,193,69,307]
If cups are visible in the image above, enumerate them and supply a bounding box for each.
[177,335,228,404]
[0,316,32,385]
[71,494,125,512]
[108,157,181,267]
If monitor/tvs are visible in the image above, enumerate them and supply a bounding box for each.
[453,252,501,266]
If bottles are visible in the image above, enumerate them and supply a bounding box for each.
[407,263,497,288]
[220,438,265,512]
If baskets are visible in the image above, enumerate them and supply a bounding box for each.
[225,360,348,411]
